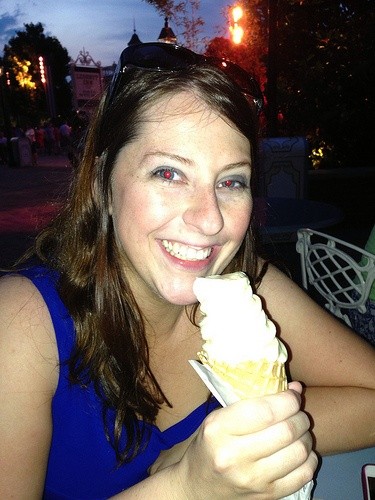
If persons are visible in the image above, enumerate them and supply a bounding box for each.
[0,42,375,500]
[0,120,82,167]
[349,226,375,349]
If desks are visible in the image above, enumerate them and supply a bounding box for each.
[245,197,345,255]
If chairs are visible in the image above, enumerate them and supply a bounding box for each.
[295,228,375,328]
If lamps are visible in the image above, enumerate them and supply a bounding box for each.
[159,15,176,41]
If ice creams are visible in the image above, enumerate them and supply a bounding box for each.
[192,271,314,499]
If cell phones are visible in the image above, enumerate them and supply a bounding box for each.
[361,464,375,500]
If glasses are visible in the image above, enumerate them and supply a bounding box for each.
[105,41,264,129]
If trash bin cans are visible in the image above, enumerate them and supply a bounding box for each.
[9,136,32,166]
